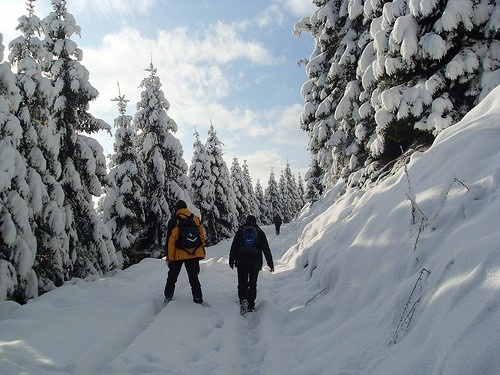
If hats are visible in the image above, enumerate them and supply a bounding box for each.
[246,215,257,224]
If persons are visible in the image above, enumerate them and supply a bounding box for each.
[228,215,274,316]
[273,213,282,235]
[164,200,206,304]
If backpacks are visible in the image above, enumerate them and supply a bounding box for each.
[172,213,202,248]
[241,224,258,248]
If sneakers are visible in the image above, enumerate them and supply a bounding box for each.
[164,297,172,303]
[240,300,255,316]
